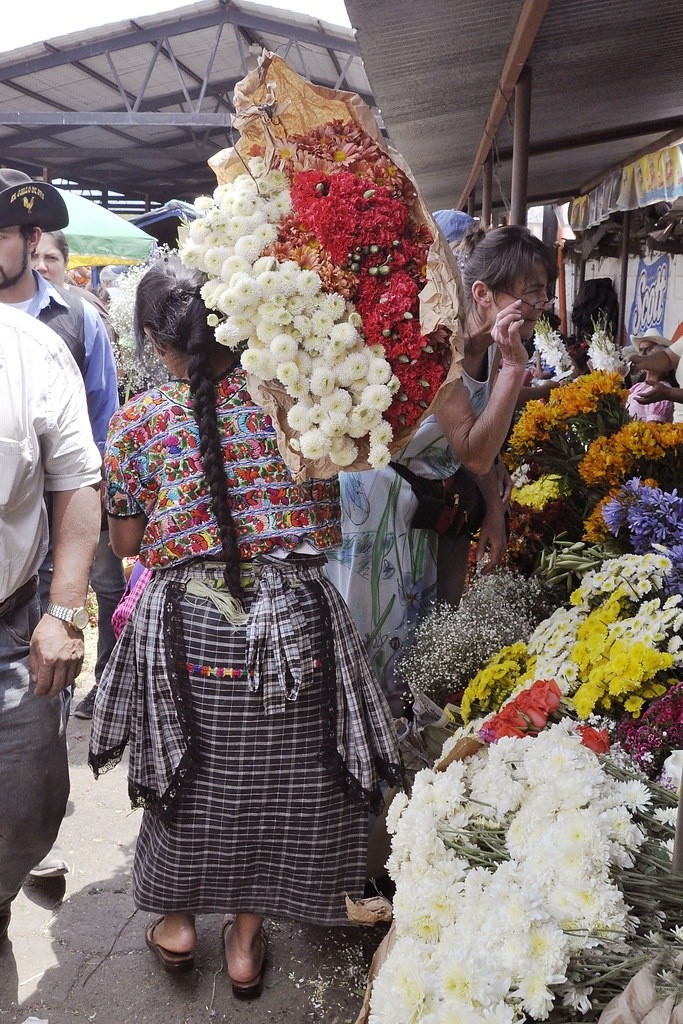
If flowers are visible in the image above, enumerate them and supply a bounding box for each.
[175,116,457,469]
[103,243,178,397]
[367,309,683,1024]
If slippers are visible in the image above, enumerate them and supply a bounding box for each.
[144,915,198,973]
[221,912,269,1001]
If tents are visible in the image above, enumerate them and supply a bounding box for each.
[54,185,158,266]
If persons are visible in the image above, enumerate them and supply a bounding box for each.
[320,225,558,899]
[0,299,104,954]
[89,256,406,998]
[0,167,156,878]
[431,208,683,511]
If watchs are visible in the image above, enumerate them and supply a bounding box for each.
[45,604,92,629]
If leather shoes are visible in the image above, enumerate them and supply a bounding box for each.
[28,856,70,877]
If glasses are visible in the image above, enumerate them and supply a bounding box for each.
[497,288,560,310]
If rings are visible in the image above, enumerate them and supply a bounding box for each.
[495,323,499,327]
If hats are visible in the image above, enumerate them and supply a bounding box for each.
[1,168,69,233]
[431,207,477,241]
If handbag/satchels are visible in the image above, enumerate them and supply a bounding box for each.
[392,461,486,540]
